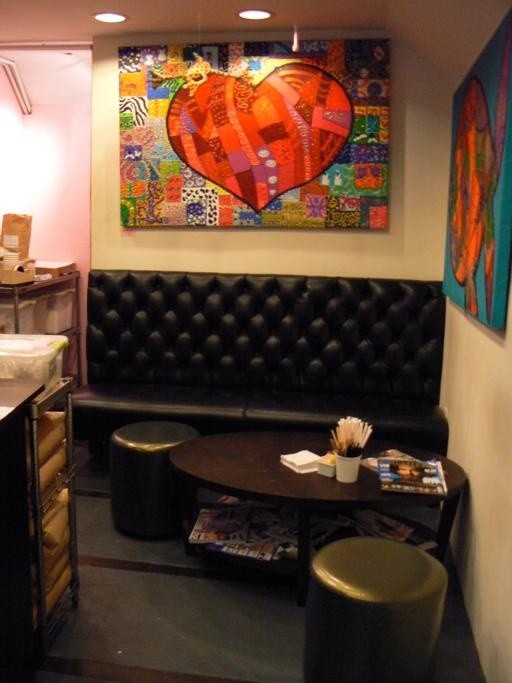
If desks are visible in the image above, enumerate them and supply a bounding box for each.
[0,381,78,683]
[169,432,467,608]
[0,270,81,438]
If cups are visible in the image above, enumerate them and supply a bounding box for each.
[3,251,21,271]
[333,450,362,485]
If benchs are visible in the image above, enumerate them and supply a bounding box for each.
[70,268,449,479]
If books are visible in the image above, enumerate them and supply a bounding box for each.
[188,449,448,562]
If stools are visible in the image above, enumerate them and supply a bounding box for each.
[301,536,449,683]
[109,421,199,540]
